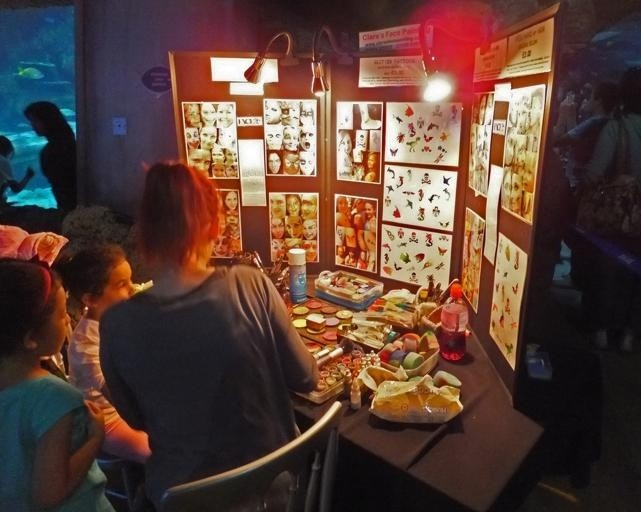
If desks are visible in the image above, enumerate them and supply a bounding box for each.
[278,275,548,510]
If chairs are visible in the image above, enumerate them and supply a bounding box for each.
[162,398,348,512]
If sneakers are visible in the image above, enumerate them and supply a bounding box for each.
[593,330,635,352]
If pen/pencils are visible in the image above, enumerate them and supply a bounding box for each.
[426,281,441,302]
[253,250,285,276]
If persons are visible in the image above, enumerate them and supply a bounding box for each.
[546,59,641,354]
[183,102,244,259]
[1,255,120,511]
[22,101,78,211]
[501,90,543,224]
[100,159,322,505]
[57,242,154,465]
[0,135,36,220]
[267,100,382,274]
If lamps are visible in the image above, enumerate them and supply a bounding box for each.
[241,15,483,106]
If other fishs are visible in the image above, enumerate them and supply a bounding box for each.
[59,108,75,116]
[12,67,45,79]
[0,121,49,152]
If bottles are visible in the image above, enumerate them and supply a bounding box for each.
[440,283,469,361]
[288,248,307,304]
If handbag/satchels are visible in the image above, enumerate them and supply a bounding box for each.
[576,173,641,237]
